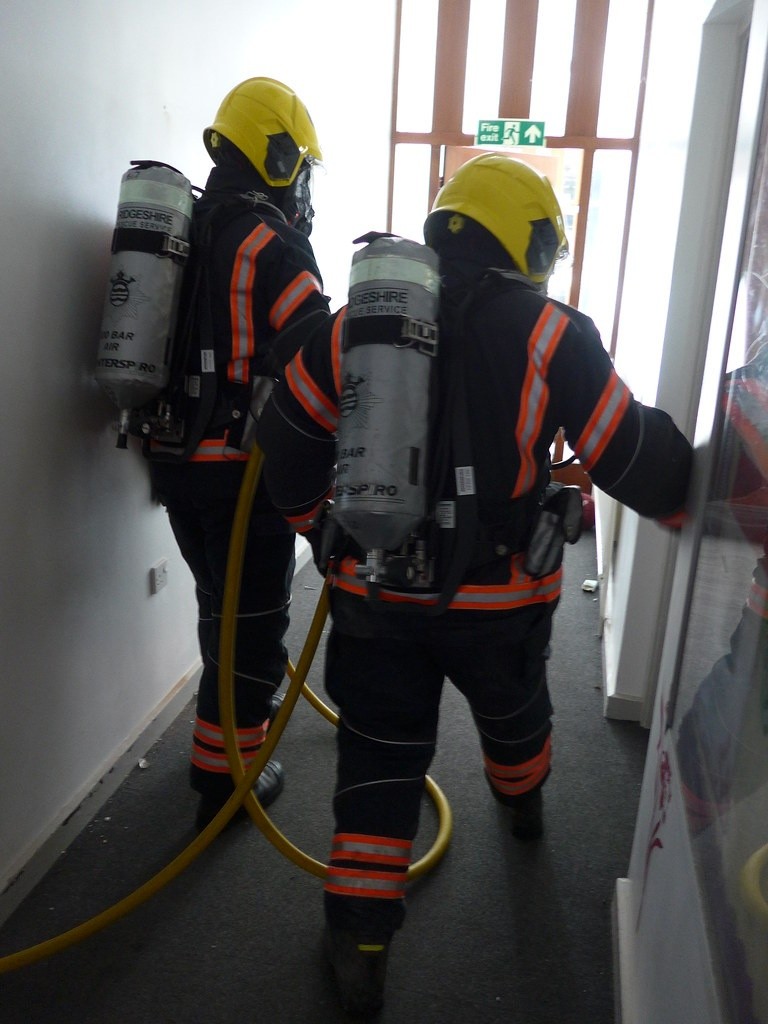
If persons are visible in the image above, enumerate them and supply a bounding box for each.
[250,154,694,966]
[146,76,332,813]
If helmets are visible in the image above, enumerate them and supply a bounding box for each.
[201,75,323,188]
[422,153,569,283]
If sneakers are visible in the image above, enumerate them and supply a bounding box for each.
[499,786,547,845]
[269,691,289,721]
[198,759,285,833]
[321,918,389,1018]
